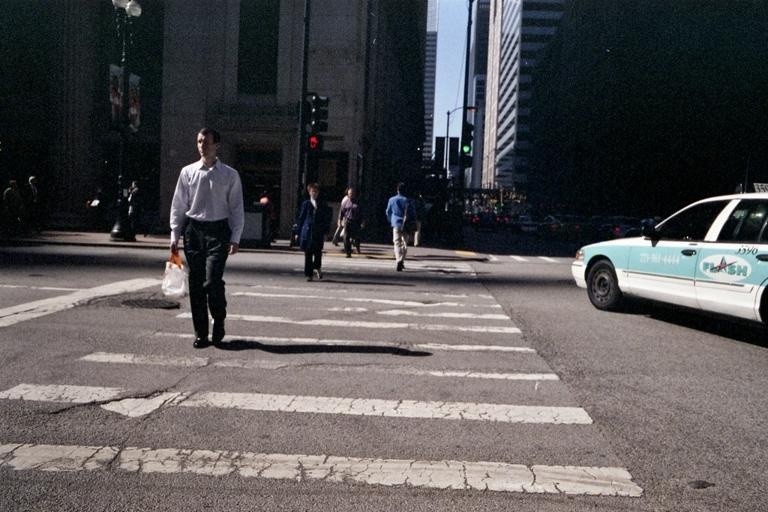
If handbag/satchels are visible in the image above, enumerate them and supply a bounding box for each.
[161,253,189,302]
[400,203,417,232]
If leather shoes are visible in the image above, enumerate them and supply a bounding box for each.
[193,336,209,349]
[211,318,225,343]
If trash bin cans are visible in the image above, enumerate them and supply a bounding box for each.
[239,205,271,250]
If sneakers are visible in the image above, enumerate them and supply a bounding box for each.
[303,268,323,281]
[396,261,406,271]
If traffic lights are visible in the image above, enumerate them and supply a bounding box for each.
[309,97,331,152]
[462,122,473,158]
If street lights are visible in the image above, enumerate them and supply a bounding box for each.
[110,1,142,242]
[444,105,480,215]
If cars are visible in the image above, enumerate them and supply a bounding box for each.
[489,212,665,245]
[571,182,768,335]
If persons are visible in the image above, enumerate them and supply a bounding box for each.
[4,175,40,235]
[127,180,140,217]
[169,128,244,348]
[296,182,329,281]
[385,183,417,271]
[332,186,360,258]
[128,86,140,132]
[109,75,122,126]
